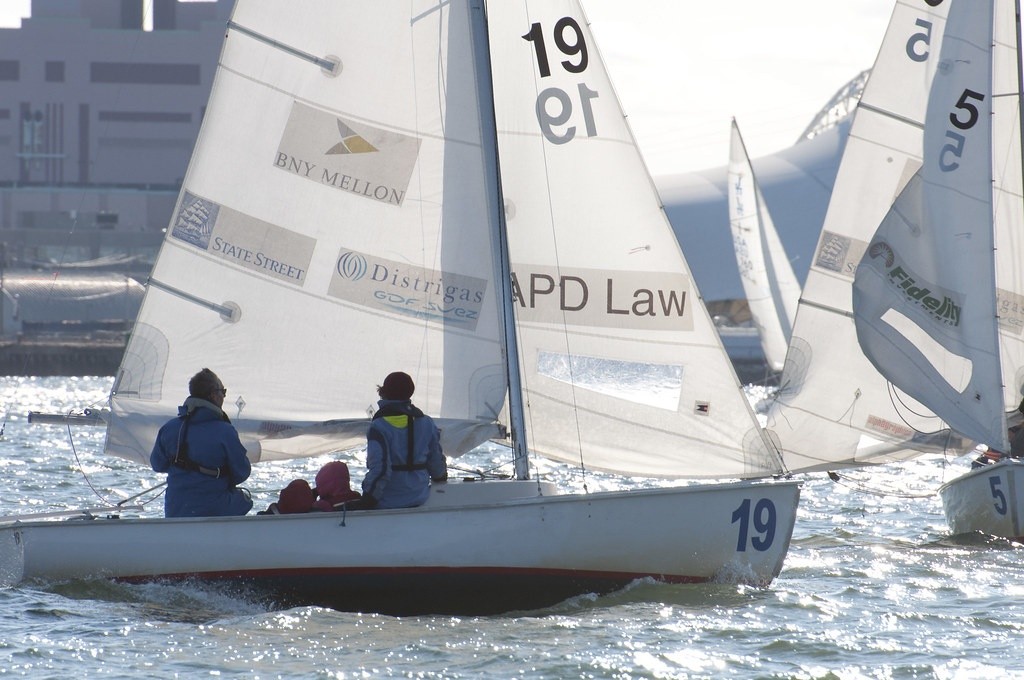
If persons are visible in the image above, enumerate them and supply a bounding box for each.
[149,367,252,517]
[360,372,449,510]
[307,461,362,513]
[255,479,314,515]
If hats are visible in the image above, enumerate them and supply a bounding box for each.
[384,372,414,398]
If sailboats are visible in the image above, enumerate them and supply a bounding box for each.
[740,0,1024,541]
[725,116,803,413]
[0,0,800,616]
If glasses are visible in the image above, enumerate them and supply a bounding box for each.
[215,388,227,395]
